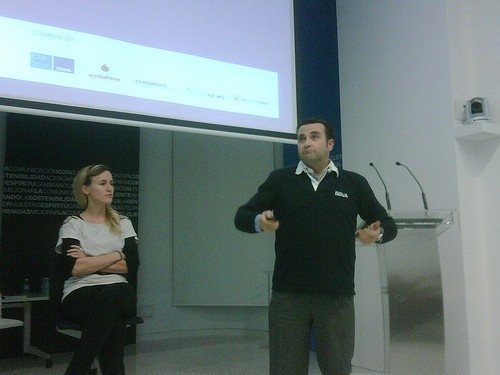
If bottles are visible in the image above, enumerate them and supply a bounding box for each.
[40,277,49,296]
[23,278,30,297]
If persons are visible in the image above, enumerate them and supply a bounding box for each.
[53,164,138,375]
[233,119,398,375]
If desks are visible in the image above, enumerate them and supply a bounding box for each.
[0,295,53,369]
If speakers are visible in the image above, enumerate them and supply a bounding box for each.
[466,96,489,121]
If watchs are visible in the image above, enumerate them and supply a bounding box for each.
[375,229,383,243]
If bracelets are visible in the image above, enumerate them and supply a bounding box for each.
[114,251,122,261]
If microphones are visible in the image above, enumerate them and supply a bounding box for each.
[368,162,391,210]
[395,161,428,211]
